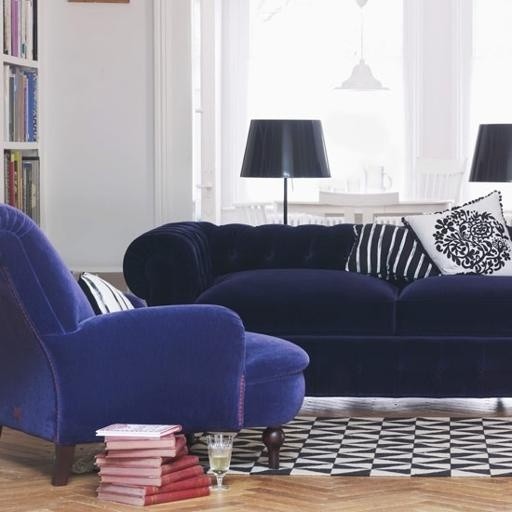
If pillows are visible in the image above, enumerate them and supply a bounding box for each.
[345,223,447,281]
[402,191,512,276]
[80,273,134,317]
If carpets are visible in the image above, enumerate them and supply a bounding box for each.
[187,415,512,478]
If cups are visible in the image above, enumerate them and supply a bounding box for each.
[207,433,237,491]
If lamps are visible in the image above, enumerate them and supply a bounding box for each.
[240,119,331,226]
[468,124,512,183]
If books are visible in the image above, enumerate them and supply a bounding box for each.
[4,0,34,60]
[96,425,211,507]
[4,149,40,226]
[4,64,33,142]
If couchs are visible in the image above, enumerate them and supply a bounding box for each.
[0,204,310,486]
[122,221,510,398]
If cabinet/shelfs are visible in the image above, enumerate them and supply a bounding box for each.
[0,0,42,231]
